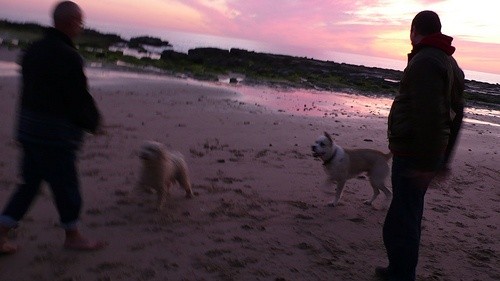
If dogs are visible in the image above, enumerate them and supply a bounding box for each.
[311,131,392,209]
[135,141,194,209]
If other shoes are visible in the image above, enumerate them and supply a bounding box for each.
[374,265,416,281]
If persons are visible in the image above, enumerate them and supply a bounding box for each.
[374,9,466,281]
[0,1,111,255]
